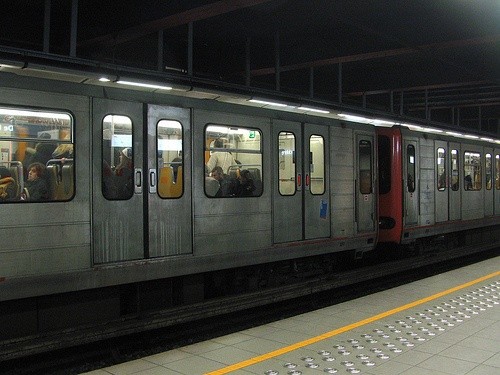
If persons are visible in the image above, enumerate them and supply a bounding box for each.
[103,148,133,199]
[440,173,473,190]
[158,136,181,183]
[204,138,255,197]
[0,166,17,202]
[14,132,73,163]
[22,162,51,201]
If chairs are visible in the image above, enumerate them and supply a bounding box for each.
[0,159,73,201]
[226,164,262,180]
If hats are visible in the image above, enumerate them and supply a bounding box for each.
[119,147,133,159]
[214,137,233,146]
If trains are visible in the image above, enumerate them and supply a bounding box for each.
[0,44,500,302]
